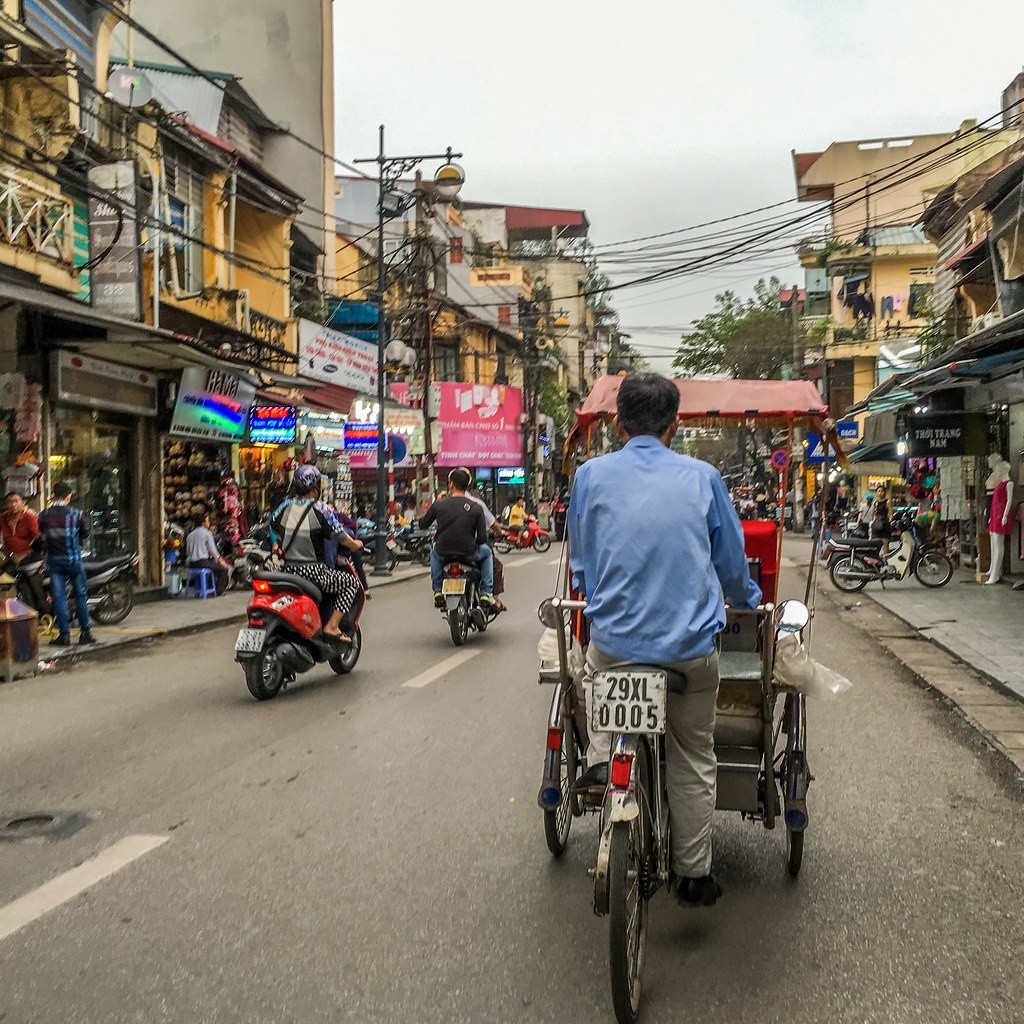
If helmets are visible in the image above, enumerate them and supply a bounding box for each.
[294,465,322,489]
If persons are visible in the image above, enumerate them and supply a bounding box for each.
[984,462,1014,586]
[804,493,831,554]
[857,495,876,525]
[835,479,849,516]
[384,499,404,532]
[569,370,762,910]
[37,482,96,648]
[186,512,233,599]
[509,496,537,549]
[873,486,893,554]
[419,466,510,612]
[735,480,795,531]
[0,491,57,626]
[538,481,571,541]
[981,454,1002,577]
[269,465,365,642]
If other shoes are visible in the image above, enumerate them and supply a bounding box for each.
[218,592,227,596]
[50,635,71,646]
[81,632,96,644]
[515,542,522,547]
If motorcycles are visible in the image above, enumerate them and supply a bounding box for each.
[490,514,552,554]
[425,531,507,647]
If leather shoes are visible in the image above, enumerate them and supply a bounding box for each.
[675,873,721,907]
[573,766,610,792]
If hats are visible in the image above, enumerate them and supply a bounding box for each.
[867,495,873,501]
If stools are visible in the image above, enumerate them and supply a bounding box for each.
[186,568,218,600]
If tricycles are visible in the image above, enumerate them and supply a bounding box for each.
[538,372,854,1022]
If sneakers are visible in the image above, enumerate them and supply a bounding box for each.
[434,592,446,607]
[479,594,493,608]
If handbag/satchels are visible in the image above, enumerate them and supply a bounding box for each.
[871,518,884,531]
[263,552,286,572]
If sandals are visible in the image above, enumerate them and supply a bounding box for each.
[494,600,507,611]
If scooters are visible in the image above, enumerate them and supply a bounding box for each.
[729,487,784,527]
[232,544,373,700]
[354,522,433,573]
[12,536,142,628]
[235,513,286,593]
[820,502,954,592]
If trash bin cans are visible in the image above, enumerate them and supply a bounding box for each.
[0,595,41,682]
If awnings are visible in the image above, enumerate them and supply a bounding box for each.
[863,407,992,458]
[850,440,904,467]
[0,279,257,392]
[846,461,901,477]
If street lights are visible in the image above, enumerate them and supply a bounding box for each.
[372,341,419,577]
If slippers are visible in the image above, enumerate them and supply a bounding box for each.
[322,631,353,645]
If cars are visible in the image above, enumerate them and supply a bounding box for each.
[551,488,573,543]
